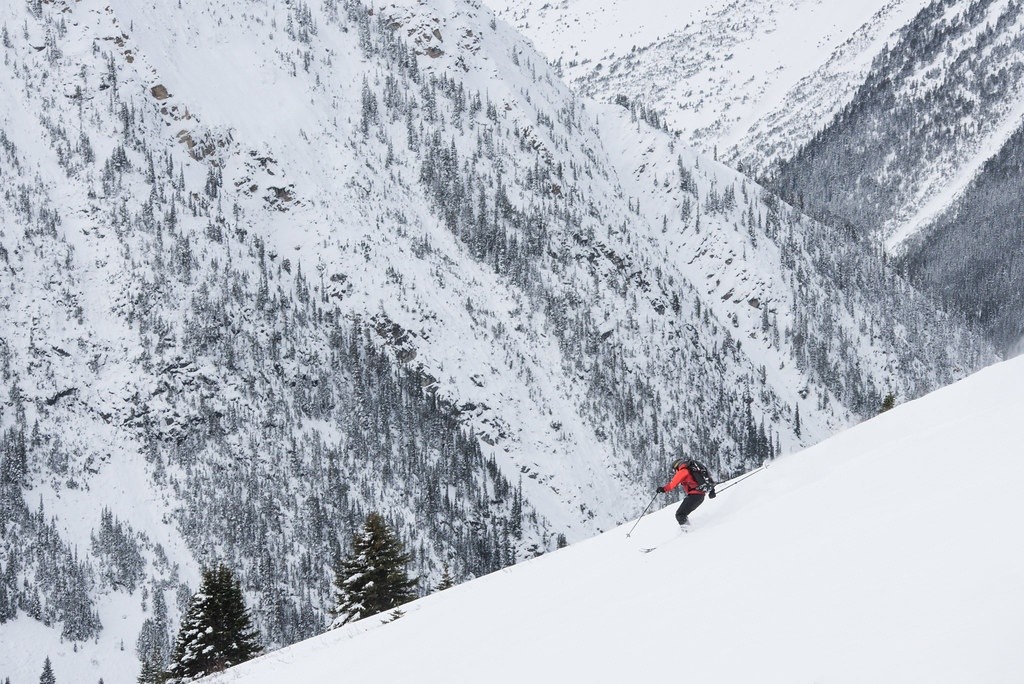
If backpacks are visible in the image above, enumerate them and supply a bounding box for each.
[686,458,714,492]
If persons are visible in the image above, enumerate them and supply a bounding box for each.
[655,458,717,532]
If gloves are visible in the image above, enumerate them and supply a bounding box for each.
[657,487,665,493]
[709,489,716,498]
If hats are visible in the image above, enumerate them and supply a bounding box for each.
[672,459,683,469]
